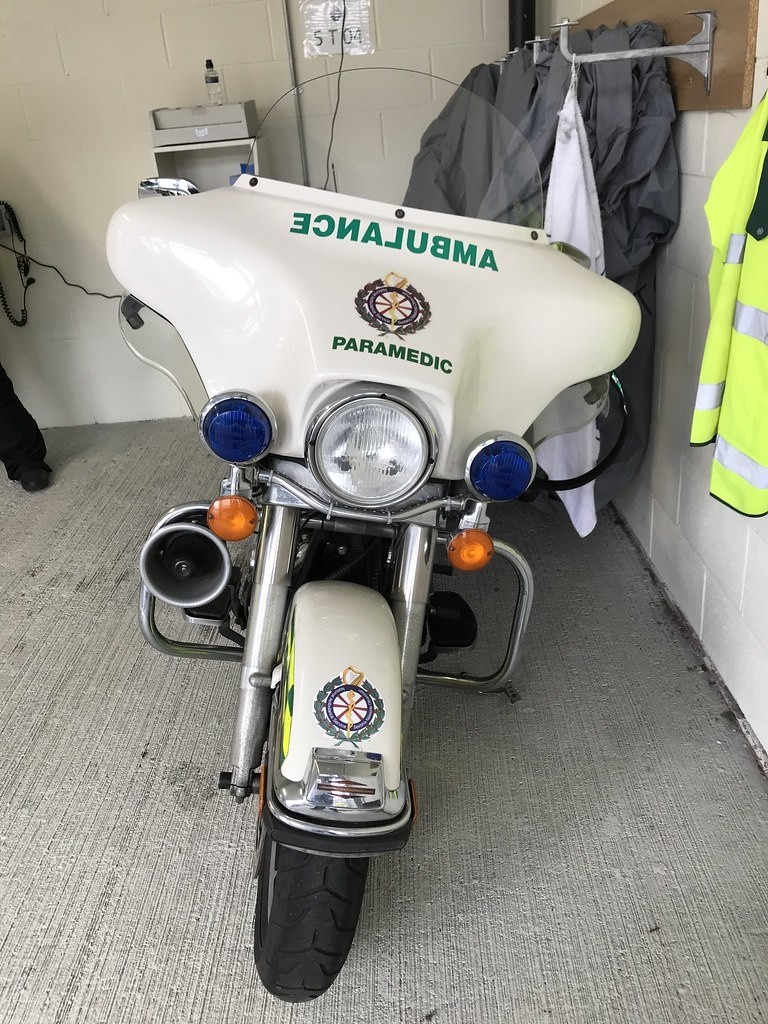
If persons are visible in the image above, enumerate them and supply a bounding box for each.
[0,364,54,492]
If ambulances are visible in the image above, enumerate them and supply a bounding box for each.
[106,62,644,1000]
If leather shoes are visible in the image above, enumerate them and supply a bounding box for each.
[19,469,51,490]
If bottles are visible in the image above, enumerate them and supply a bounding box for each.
[203,58,223,108]
